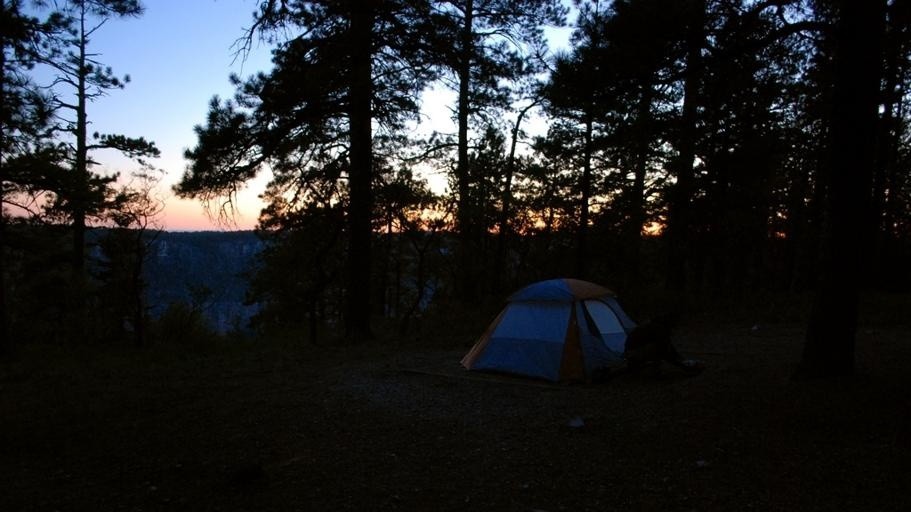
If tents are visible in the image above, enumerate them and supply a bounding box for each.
[455,277,644,390]
